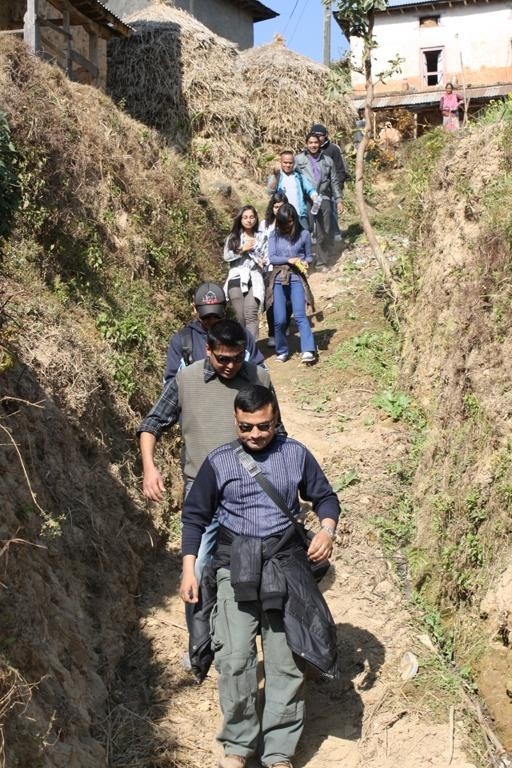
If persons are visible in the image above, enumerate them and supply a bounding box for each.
[130,321,287,686]
[439,82,465,126]
[220,124,346,364]
[178,385,342,768]
[163,281,266,385]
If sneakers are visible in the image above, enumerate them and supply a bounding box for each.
[300,352,316,362]
[268,759,294,768]
[315,264,329,272]
[268,336,277,347]
[275,354,289,362]
[218,753,247,767]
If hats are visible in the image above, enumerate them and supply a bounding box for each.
[193,281,228,317]
[312,124,326,135]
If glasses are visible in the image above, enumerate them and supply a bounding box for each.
[235,417,275,432]
[212,352,246,363]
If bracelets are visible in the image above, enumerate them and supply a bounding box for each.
[323,526,335,538]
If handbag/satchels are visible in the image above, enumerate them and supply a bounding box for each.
[302,530,330,583]
